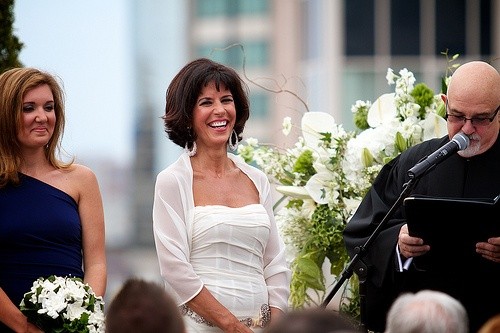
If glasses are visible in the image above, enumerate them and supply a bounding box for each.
[445,99,500,126]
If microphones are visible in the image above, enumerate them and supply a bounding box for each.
[407,132,471,180]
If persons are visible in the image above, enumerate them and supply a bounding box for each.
[0,67,107,333]
[385,290,468,333]
[106,280,185,333]
[341,60,500,333]
[152,58,292,333]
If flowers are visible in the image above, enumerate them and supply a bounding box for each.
[237,48,460,326]
[19,274,107,333]
